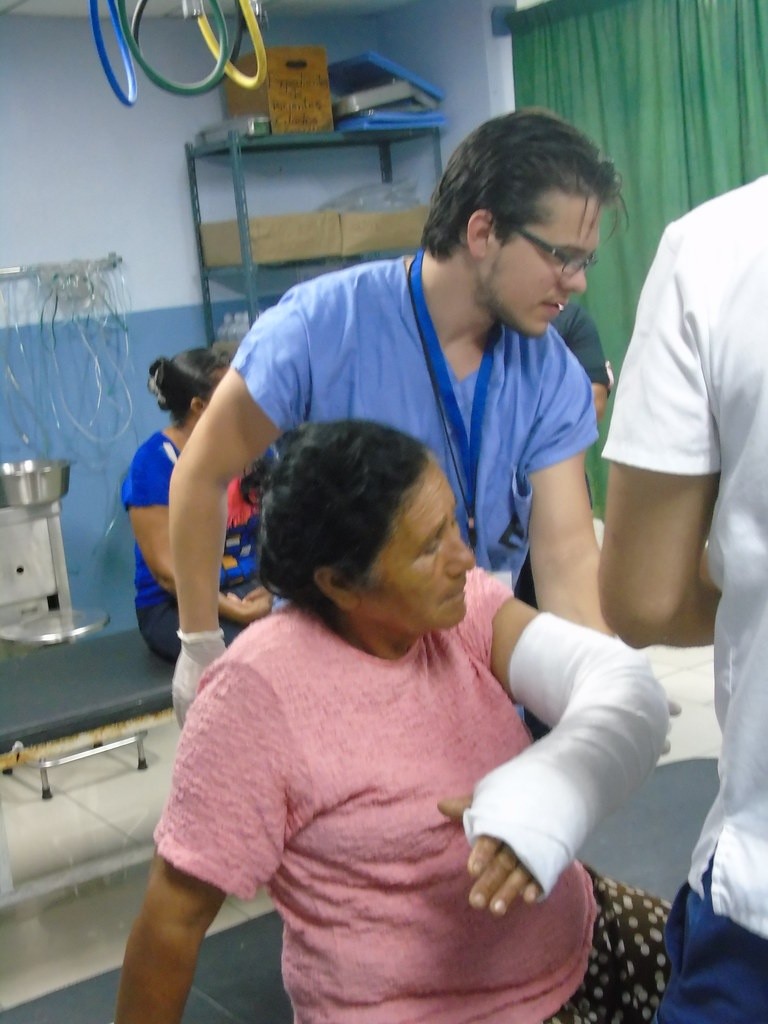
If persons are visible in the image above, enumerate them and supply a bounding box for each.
[601,176,767,1023]
[508,302,614,741]
[170,108,679,754]
[122,348,277,664]
[117,417,675,1024]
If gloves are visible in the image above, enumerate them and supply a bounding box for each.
[172,626,229,728]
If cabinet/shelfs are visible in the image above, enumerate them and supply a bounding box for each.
[184,127,449,351]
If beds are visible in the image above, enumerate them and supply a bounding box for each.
[0,626,174,799]
[0,758,720,1024]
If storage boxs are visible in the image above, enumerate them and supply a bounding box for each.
[199,209,430,268]
[227,47,335,136]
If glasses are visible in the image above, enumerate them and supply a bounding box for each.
[202,348,228,376]
[511,225,598,279]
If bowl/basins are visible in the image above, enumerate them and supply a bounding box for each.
[0,458,71,508]
